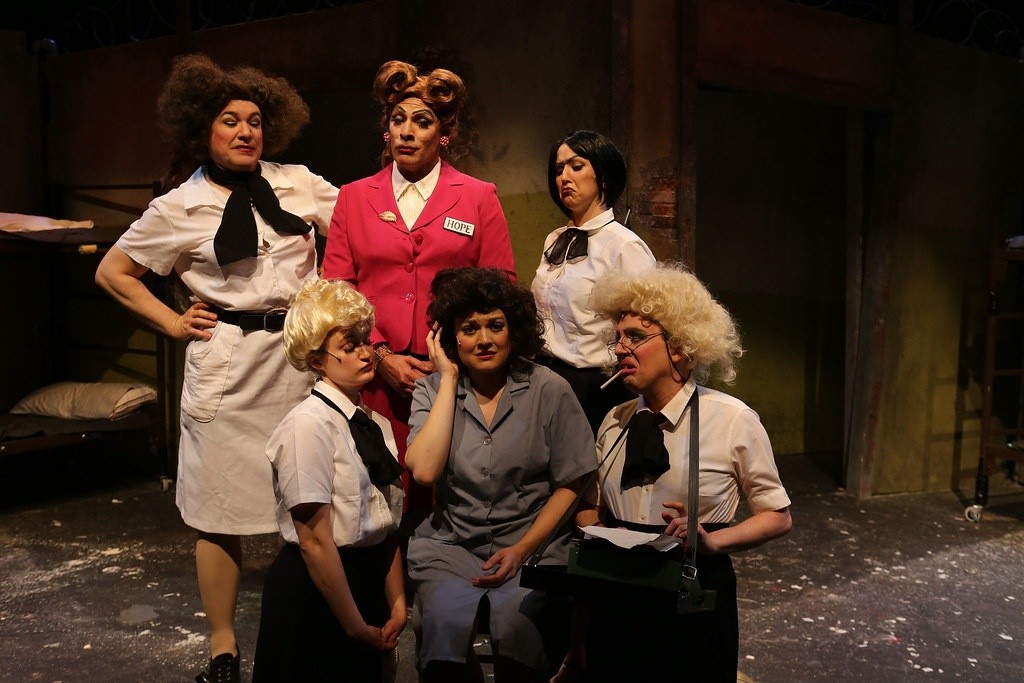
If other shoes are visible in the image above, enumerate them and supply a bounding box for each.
[210,644,240,683]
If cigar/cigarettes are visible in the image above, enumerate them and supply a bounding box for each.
[600,369,623,389]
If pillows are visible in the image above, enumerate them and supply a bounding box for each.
[10,381,157,422]
[0,212,97,254]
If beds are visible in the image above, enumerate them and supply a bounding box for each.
[974,192,1024,507]
[0,180,178,483]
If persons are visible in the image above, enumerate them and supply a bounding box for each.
[92,54,344,683]
[571,267,794,683]
[318,58,518,549]
[531,126,661,439]
[402,265,599,683]
[251,278,407,682]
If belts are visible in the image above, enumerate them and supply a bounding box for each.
[204,302,288,332]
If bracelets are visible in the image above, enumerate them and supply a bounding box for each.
[375,344,393,372]
[592,520,603,527]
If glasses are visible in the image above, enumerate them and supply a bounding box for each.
[608,333,671,351]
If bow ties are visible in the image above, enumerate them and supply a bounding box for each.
[544,227,589,264]
[310,388,406,491]
[620,411,671,494]
[206,159,313,268]
